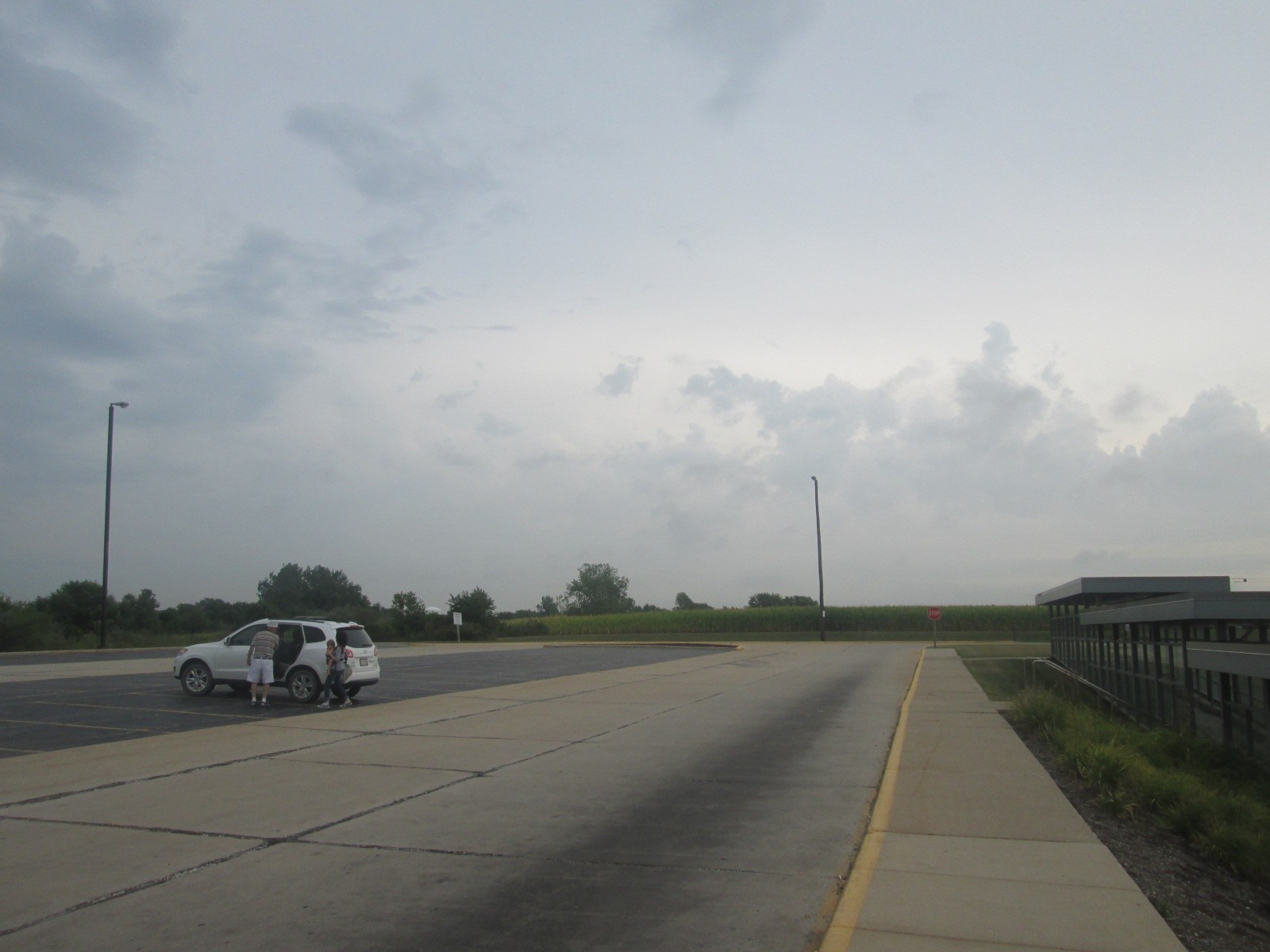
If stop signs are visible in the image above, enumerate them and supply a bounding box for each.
[927,606,941,619]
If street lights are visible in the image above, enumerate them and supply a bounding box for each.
[811,474,826,641]
[98,401,130,650]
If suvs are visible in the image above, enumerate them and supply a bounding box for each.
[171,616,380,704]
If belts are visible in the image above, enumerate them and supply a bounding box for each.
[253,656,272,660]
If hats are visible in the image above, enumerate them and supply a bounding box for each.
[266,621,279,628]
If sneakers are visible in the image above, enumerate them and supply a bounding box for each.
[317,703,329,708]
[339,700,352,708]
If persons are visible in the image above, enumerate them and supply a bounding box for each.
[246,621,280,707]
[317,632,353,708]
[326,639,337,675]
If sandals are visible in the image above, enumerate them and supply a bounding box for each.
[249,700,257,705]
[260,701,273,706]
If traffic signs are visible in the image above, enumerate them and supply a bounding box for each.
[453,612,463,625]
